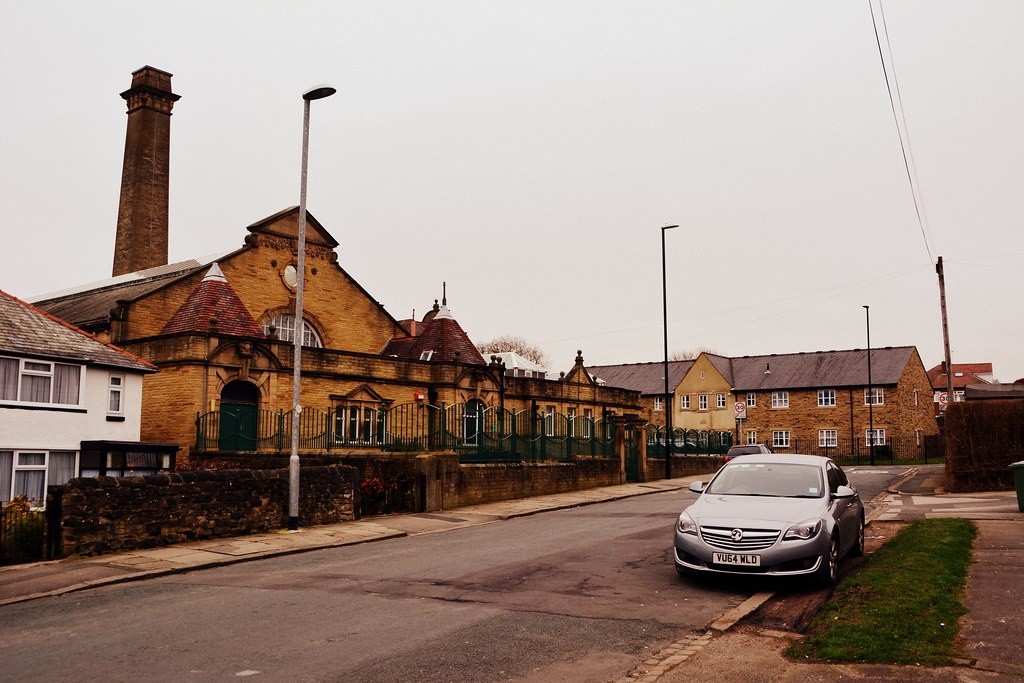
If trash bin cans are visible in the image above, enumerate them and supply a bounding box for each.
[1008,460,1024,512]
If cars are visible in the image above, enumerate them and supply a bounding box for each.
[672,453,865,593]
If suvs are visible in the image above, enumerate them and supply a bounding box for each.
[724,443,772,466]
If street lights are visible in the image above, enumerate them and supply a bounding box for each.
[661,223,680,477]
[286,85,337,530]
[863,305,873,465]
